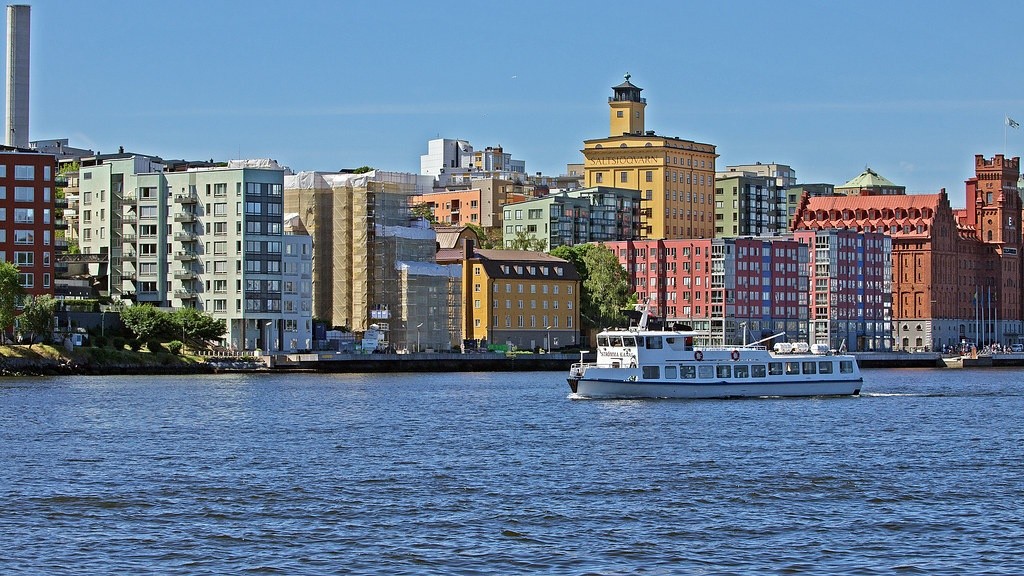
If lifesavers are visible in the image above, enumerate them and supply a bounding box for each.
[731,350,740,361]
[694,351,703,362]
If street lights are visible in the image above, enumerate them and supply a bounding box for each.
[547,325,552,353]
[266,321,272,355]
[416,322,424,352]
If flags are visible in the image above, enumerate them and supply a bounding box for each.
[971,287,997,306]
[1006,117,1020,129]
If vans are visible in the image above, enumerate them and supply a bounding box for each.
[1010,344,1024,352]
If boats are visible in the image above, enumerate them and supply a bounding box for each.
[565,296,864,398]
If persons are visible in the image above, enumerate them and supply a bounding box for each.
[374,342,398,354]
[944,342,1012,354]
